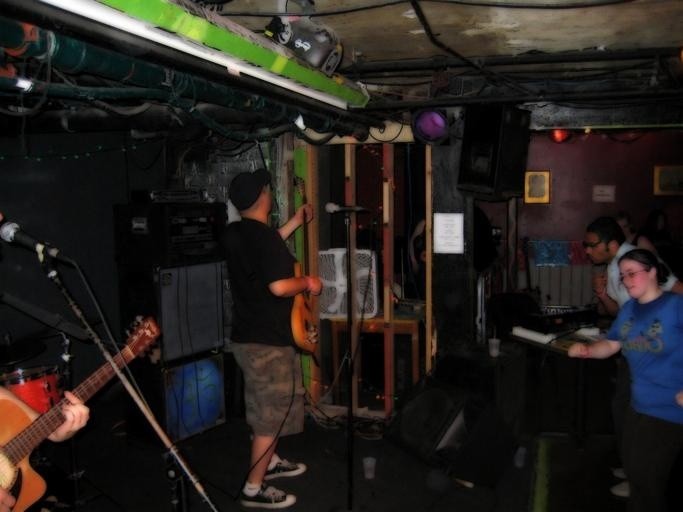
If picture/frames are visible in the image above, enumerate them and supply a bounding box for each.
[525,170,551,204]
[652,165,683,195]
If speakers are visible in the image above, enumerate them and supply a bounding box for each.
[114,262,226,363]
[455,104,532,200]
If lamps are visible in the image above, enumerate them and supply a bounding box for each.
[411,108,453,147]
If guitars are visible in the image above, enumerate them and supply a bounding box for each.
[290,177,322,354]
[1,316,161,512]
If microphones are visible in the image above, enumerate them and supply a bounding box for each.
[323,198,364,216]
[0,222,59,265]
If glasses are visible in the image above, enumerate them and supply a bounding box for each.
[582,241,600,248]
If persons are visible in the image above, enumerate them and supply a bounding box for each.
[0,212,91,512]
[408,206,683,511]
[227,168,323,508]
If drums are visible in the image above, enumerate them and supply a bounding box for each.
[1,363,61,416]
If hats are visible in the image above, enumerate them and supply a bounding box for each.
[228,168,271,211]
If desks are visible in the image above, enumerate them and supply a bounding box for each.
[328,306,422,405]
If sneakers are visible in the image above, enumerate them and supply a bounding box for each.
[241,484,296,509]
[610,480,630,497]
[613,468,627,478]
[264,458,307,480]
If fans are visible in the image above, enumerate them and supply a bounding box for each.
[318,249,378,319]
